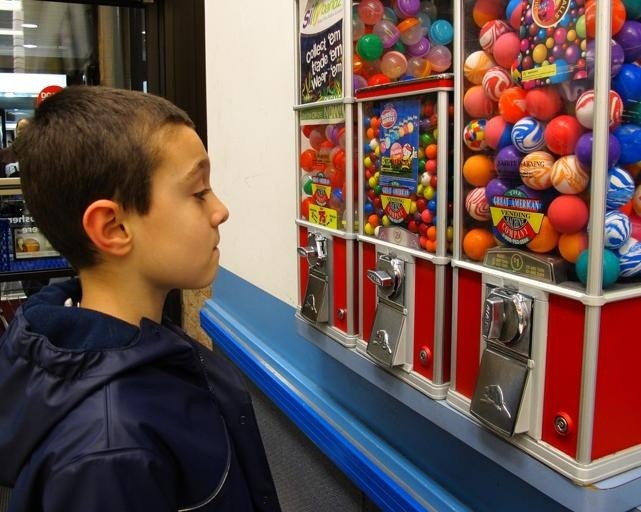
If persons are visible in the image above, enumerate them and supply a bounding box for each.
[2,85,284,510]
[14,117,33,138]
